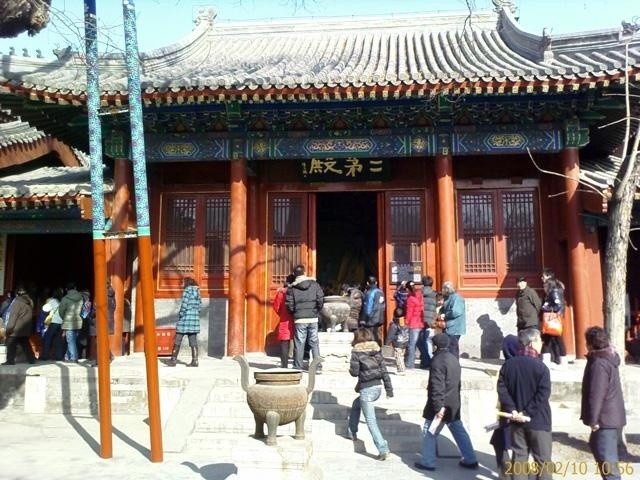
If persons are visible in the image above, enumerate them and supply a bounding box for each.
[271,265,568,373]
[413,333,479,470]
[495,333,555,480]
[0,278,131,367]
[341,325,394,461]
[577,325,631,480]
[165,277,203,367]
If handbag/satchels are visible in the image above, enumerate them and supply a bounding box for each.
[542,312,563,337]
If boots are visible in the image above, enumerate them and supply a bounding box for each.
[165,344,179,365]
[186,346,198,366]
